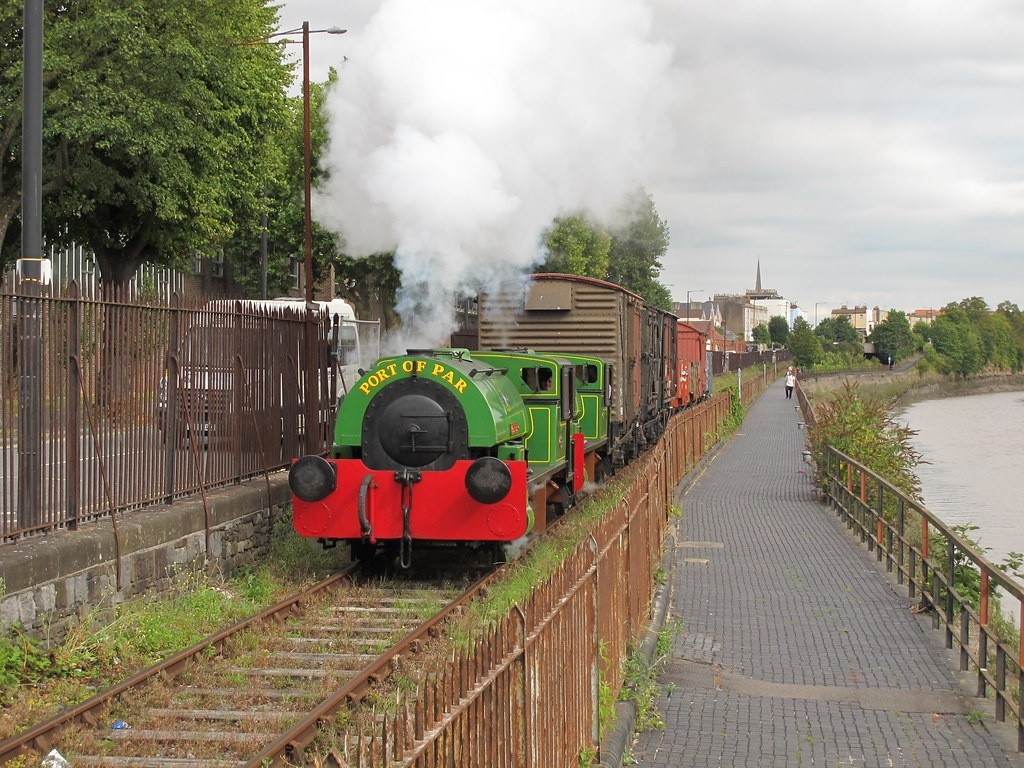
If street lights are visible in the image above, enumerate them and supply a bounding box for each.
[685,289,705,324]
[815,302,826,328]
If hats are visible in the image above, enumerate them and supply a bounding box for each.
[540,371,552,381]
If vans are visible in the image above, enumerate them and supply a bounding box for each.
[155,297,359,449]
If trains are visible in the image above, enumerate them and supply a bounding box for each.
[288,274,708,568]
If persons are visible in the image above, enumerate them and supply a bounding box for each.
[785,365,801,399]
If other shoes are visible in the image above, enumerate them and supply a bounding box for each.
[786,396,788,399]
[789,397,791,399]
